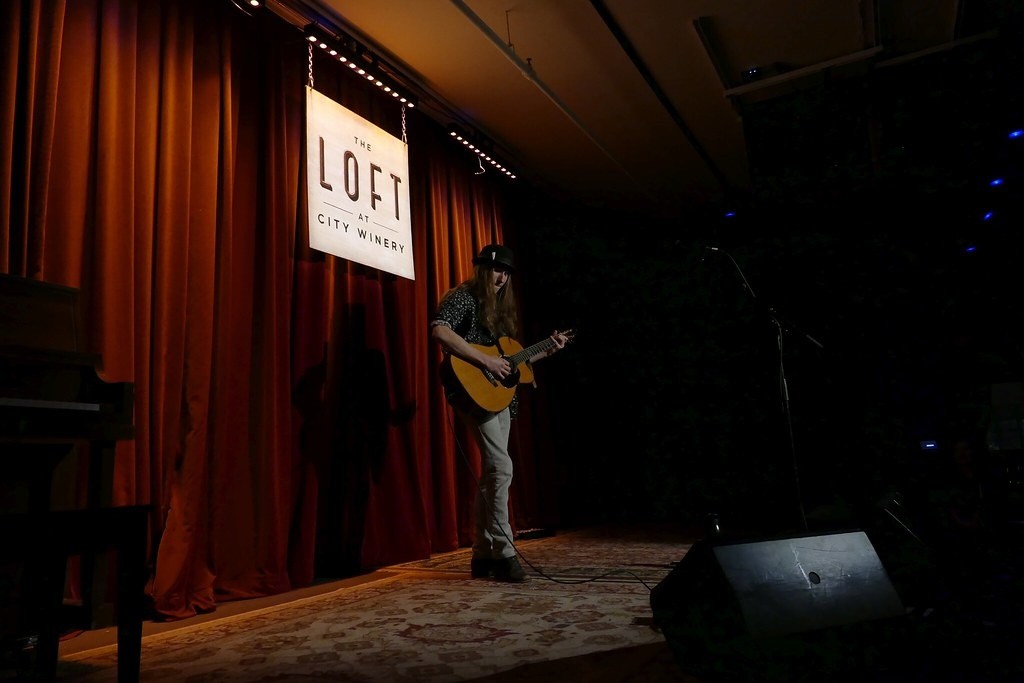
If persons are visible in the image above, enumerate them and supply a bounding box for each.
[429,243,567,582]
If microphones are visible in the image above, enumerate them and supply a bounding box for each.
[676,240,721,253]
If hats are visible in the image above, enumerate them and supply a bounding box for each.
[473,243,517,271]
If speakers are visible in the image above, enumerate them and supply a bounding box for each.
[649,530,907,683]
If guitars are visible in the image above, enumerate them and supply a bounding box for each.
[439,330,577,423]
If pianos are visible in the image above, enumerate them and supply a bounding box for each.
[0,273,157,683]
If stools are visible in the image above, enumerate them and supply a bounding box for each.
[0,504,155,683]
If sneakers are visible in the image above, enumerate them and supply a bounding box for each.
[471,556,496,576]
[493,556,531,584]
